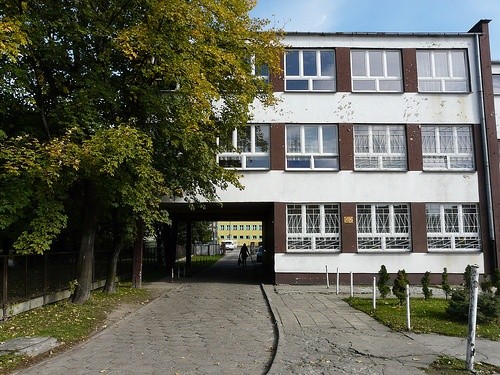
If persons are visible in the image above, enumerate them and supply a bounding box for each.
[239,243,250,268]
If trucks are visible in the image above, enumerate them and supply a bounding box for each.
[223,240,236,251]
